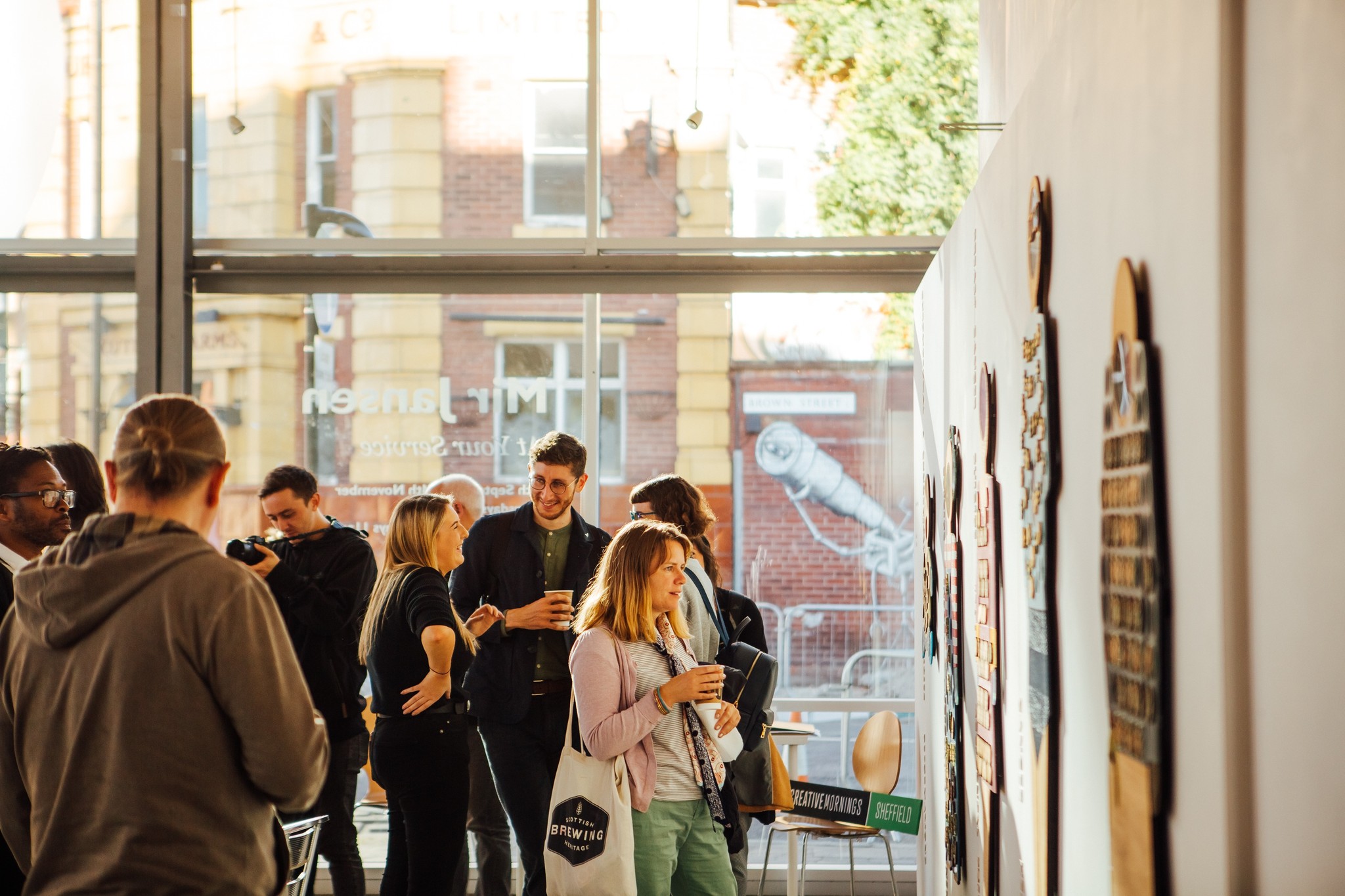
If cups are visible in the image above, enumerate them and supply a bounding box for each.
[690,665,725,709]
[544,590,574,626]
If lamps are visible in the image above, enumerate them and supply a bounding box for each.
[227,102,246,137]
[686,97,704,129]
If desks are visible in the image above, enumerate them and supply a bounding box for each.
[770,734,808,896]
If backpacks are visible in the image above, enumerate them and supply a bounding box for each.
[684,567,779,753]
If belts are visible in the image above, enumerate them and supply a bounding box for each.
[379,700,471,718]
[531,678,573,696]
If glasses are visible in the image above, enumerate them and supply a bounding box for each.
[629,511,658,520]
[528,472,579,494]
[0,490,78,510]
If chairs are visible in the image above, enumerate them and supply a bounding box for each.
[282,695,388,896]
[758,710,900,896]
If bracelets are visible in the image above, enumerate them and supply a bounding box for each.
[653,688,669,715]
[429,666,450,675]
[657,685,670,712]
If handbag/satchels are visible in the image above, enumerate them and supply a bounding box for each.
[543,746,638,896]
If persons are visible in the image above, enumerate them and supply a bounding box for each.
[43,442,109,533]
[241,430,765,896]
[0,441,76,623]
[0,392,328,896]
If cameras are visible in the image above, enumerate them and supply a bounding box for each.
[226,536,266,565]
